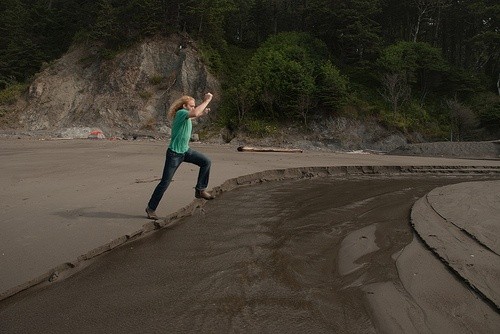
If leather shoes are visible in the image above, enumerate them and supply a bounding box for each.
[145,208,159,220]
[195,190,216,200]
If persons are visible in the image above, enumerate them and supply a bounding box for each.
[146,92,217,220]
